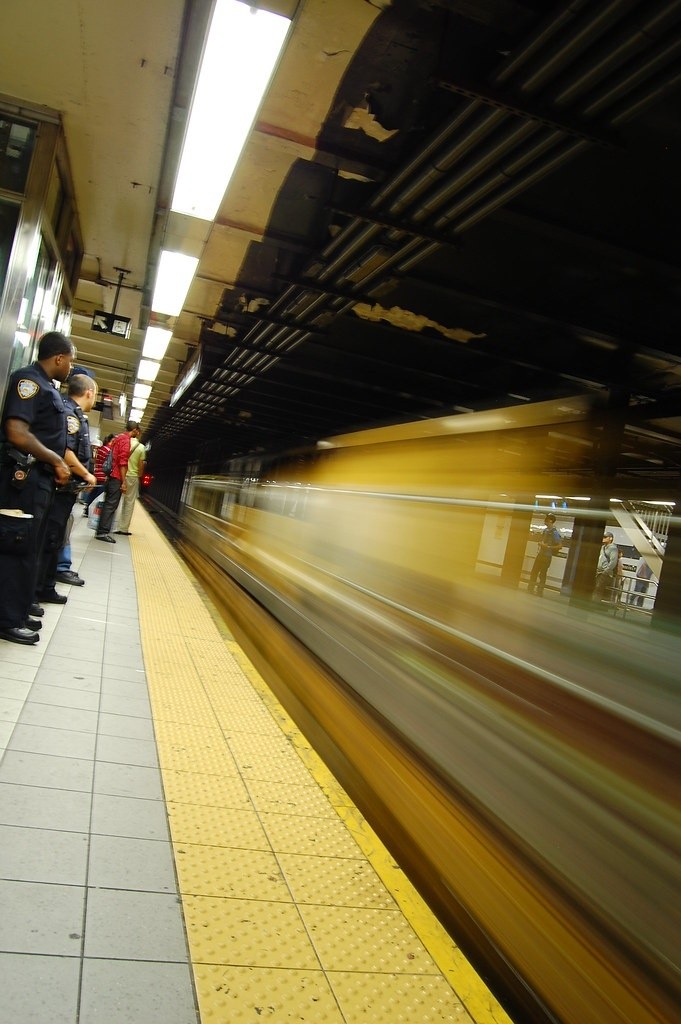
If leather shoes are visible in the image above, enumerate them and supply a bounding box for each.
[0,628,40,644]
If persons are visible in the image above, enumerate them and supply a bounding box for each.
[629,540,655,607]
[82,420,147,543]
[593,531,623,604]
[0,332,97,645]
[527,515,563,597]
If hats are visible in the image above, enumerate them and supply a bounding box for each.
[604,532,613,537]
[547,513,556,522]
[70,367,95,380]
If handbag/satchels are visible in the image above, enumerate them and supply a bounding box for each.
[88,491,122,532]
[604,545,617,576]
[636,564,652,580]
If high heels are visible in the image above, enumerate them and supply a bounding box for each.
[25,616,42,631]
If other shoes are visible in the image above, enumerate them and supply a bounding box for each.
[45,591,67,604]
[28,602,45,616]
[96,535,116,543]
[116,531,132,535]
[56,571,84,585]
[83,508,89,517]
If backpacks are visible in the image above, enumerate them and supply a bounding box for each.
[102,439,119,475]
[543,528,562,556]
